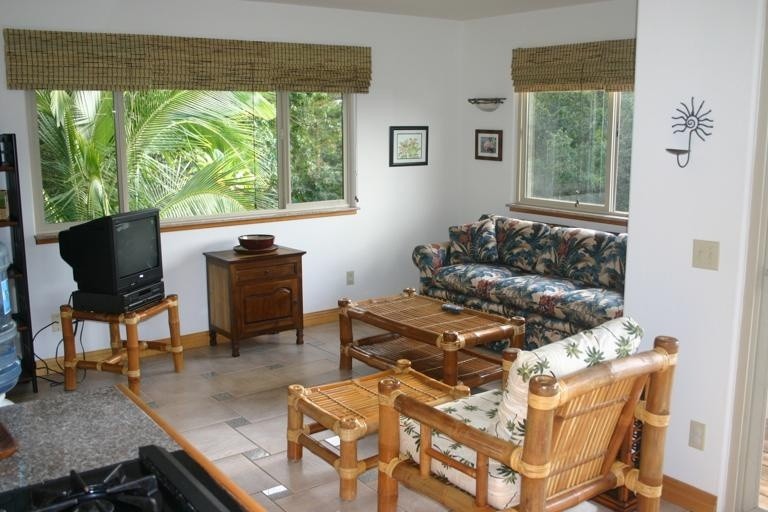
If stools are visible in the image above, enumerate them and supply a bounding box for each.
[60,294,182,395]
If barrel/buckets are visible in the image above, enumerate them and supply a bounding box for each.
[1,242,23,395]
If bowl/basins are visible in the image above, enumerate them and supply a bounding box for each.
[238,233,275,248]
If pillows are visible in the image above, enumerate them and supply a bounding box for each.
[488,316,643,464]
[449,218,498,264]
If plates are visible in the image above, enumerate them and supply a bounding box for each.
[233,244,280,254]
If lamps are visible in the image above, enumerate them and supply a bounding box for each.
[468,97,505,112]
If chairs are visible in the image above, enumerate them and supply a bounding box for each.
[377,335,679,512]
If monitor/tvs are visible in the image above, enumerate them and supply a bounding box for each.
[57,210,163,294]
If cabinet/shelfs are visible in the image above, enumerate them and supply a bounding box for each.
[0,133,38,392]
[203,243,307,357]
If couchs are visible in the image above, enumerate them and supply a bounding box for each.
[413,212,627,351]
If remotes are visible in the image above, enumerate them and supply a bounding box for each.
[442,302,464,313]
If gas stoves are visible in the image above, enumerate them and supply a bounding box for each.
[0,443,249,512]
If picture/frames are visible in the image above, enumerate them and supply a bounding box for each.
[475,129,503,161]
[389,126,429,167]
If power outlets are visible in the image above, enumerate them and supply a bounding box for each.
[51,313,62,332]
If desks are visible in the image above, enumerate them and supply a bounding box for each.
[0,383,269,512]
